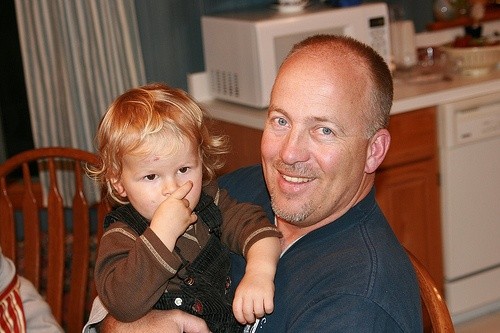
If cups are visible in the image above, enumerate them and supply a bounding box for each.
[186,72,211,104]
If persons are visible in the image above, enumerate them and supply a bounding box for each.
[82,35,423,333]
[85,82,283,333]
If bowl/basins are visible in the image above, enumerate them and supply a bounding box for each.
[441,46,500,76]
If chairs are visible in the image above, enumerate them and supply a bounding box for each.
[1,148,121,333]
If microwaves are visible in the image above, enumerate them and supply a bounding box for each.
[201,3,395,108]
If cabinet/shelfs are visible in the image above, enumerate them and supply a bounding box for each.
[193,44,500,333]
